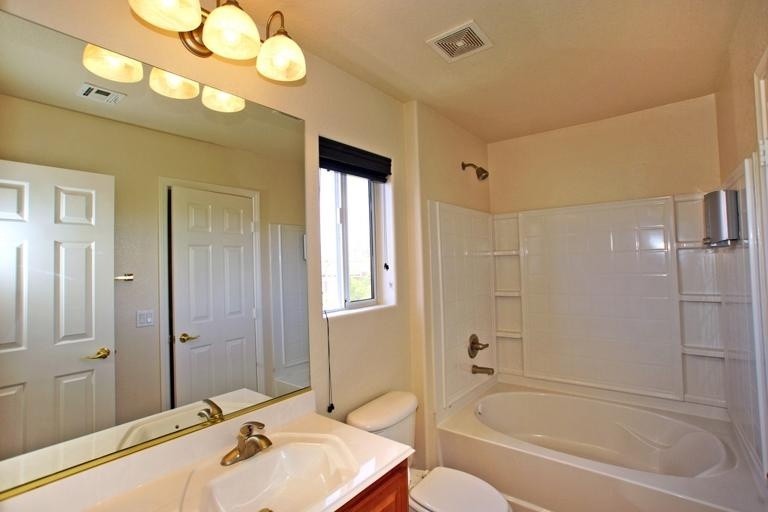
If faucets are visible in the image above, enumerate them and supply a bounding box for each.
[195,397,223,421]
[237,420,273,458]
[471,364,495,375]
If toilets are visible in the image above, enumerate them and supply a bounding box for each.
[347,388,515,511]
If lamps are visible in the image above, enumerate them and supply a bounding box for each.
[461,161,489,182]
[128,0,307,86]
[81,42,247,113]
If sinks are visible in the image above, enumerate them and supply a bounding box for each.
[116,401,254,451]
[180,430,364,512]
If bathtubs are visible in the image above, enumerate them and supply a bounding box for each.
[438,380,766,511]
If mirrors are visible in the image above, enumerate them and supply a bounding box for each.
[0,7,312,499]
